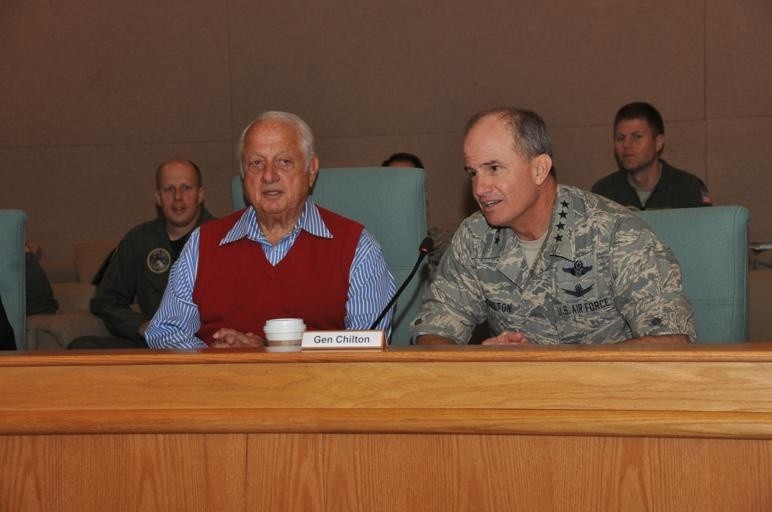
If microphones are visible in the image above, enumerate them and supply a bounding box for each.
[367,237,433,329]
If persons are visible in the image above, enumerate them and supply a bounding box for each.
[145,110,396,352]
[26,241,60,330]
[67,161,217,348]
[410,108,699,347]
[1,300,17,351]
[379,152,453,272]
[591,100,710,209]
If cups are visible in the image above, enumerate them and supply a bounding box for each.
[262,317,307,352]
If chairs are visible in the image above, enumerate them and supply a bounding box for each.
[630,206,753,344]
[231,166,431,348]
[0,207,29,350]
